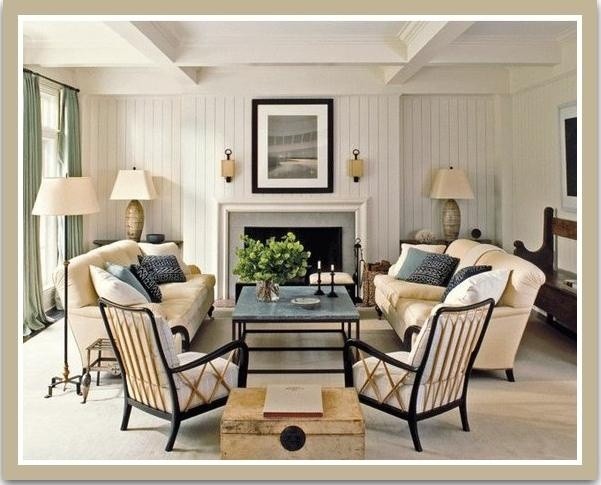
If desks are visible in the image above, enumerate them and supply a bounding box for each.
[93,239,184,251]
[400,238,491,256]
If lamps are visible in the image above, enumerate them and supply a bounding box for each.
[30,174,101,397]
[109,166,158,241]
[429,165,475,243]
[221,149,236,182]
[347,148,364,182]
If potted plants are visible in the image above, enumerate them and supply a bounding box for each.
[232,232,311,302]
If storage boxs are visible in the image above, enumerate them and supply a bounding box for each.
[221,386,365,460]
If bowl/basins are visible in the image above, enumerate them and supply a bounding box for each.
[146,233,164,244]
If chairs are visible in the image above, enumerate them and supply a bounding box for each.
[96,296,249,451]
[343,297,494,451]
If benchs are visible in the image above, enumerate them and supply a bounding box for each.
[515,205,577,346]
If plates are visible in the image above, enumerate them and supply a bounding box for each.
[289,297,320,306]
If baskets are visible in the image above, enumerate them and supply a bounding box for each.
[362,259,390,308]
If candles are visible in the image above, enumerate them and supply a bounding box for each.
[330,264,334,271]
[317,260,321,270]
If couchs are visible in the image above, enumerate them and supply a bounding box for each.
[309,272,355,306]
[52,240,215,383]
[373,239,545,381]
[236,273,279,304]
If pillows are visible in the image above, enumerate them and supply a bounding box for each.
[127,265,162,303]
[443,270,513,310]
[105,307,181,389]
[104,262,152,303]
[406,252,460,286]
[137,255,186,283]
[395,249,448,280]
[137,242,190,277]
[388,243,447,278]
[400,304,484,384]
[442,265,492,302]
[89,264,149,307]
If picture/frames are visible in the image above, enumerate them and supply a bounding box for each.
[252,99,333,193]
